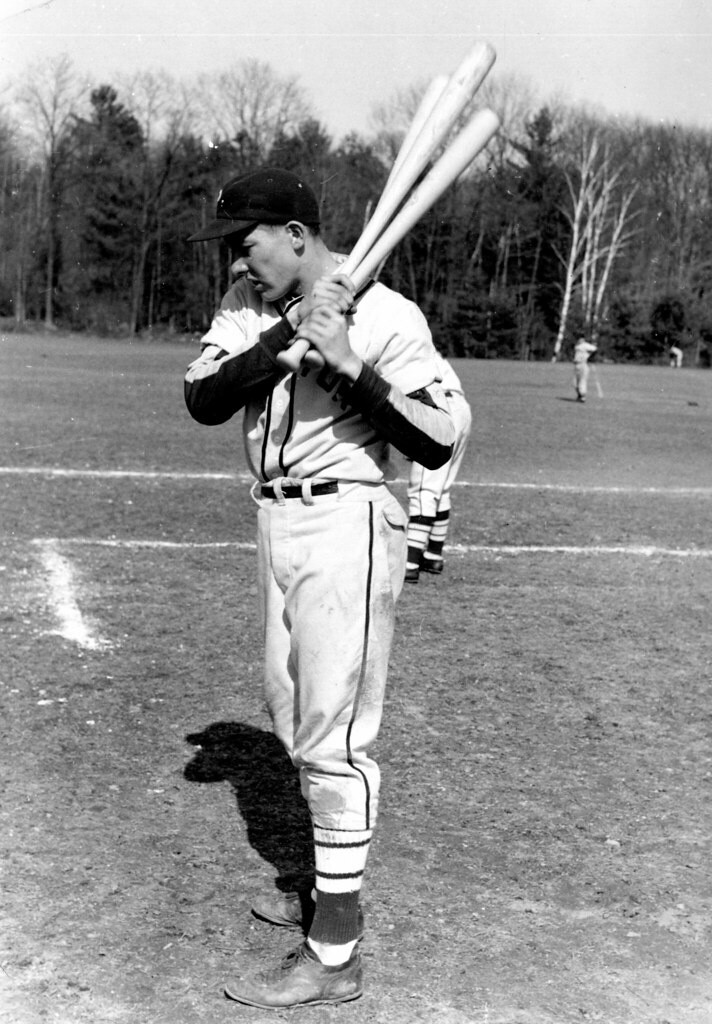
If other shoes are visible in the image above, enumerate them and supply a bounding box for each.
[577,395,586,402]
[403,562,420,583]
[420,551,444,573]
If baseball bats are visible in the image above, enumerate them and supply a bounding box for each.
[275,39,512,377]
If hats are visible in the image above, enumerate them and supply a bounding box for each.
[187,165,320,241]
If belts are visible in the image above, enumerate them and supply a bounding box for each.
[261,480,338,500]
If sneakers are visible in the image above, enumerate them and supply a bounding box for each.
[252,889,364,935]
[225,939,364,1009]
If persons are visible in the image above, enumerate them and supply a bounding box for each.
[668,344,684,369]
[573,334,599,403]
[404,351,471,585]
[185,169,452,1011]
